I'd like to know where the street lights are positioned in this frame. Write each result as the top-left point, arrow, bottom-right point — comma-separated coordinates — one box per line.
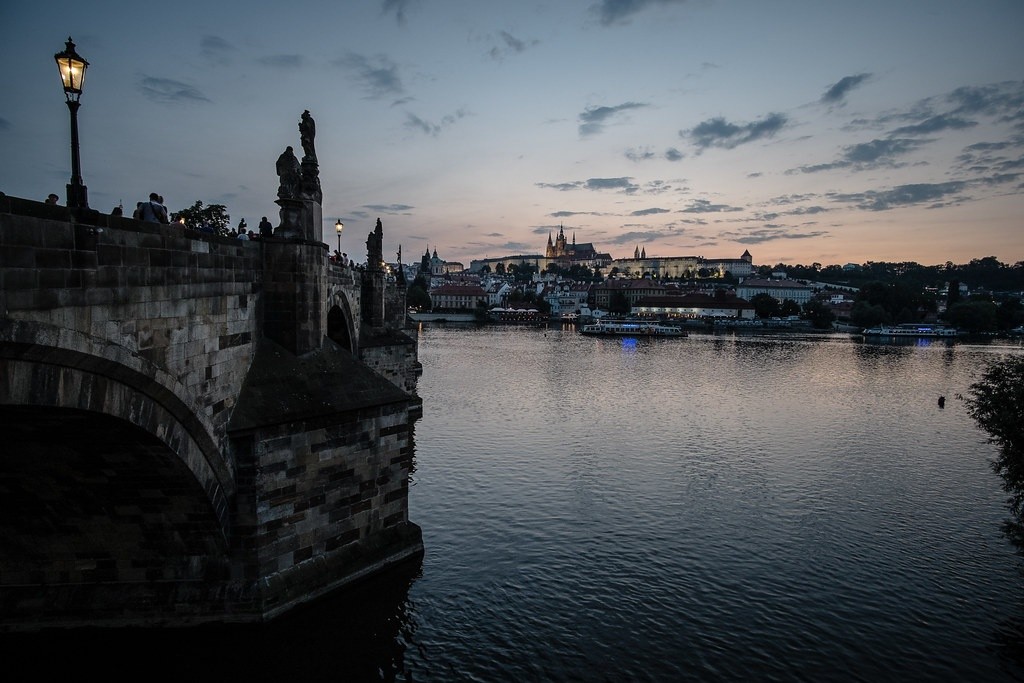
54,35 -> 90,205
334,218 -> 343,256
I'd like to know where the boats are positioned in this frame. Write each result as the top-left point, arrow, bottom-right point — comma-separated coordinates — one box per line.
833,322 -> 858,331
863,323 -> 958,336
583,319 -> 685,336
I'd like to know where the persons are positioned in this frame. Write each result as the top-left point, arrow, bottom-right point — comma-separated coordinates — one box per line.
112,207 -> 122,217
200,222 -> 214,234
229,218 -> 246,237
259,217 -> 272,237
45,194 -> 59,204
333,250 -> 364,270
276,110 -> 319,178
237,229 -> 257,240
374,218 -> 382,233
133,193 -> 182,224
